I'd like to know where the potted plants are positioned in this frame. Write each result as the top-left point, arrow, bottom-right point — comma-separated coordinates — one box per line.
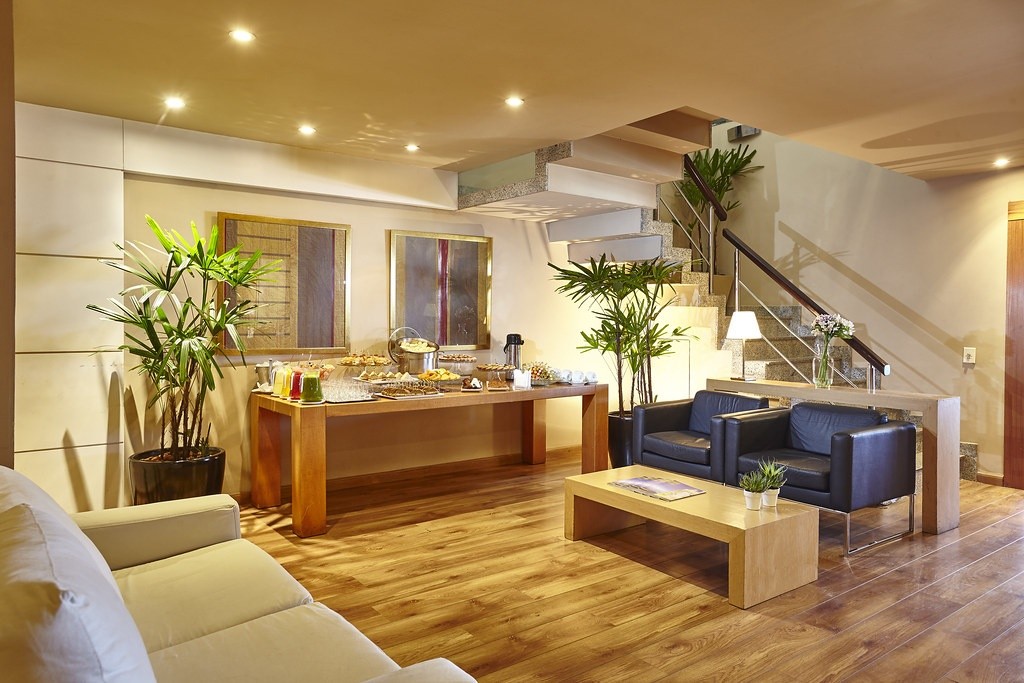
739,470 -> 766,511
757,456 -> 788,507
672,144 -> 765,307
544,255 -> 704,468
86,212 -> 283,504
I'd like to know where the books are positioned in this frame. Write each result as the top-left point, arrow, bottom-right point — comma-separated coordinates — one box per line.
608,476 -> 706,502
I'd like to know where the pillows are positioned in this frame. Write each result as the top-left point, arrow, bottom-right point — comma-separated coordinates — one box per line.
0,465 -> 157,683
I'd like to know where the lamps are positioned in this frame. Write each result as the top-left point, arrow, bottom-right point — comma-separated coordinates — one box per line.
725,310 -> 762,381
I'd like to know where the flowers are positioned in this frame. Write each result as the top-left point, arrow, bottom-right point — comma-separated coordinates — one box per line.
810,311 -> 856,349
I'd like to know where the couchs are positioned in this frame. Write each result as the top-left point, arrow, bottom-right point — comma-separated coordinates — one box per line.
725,401 -> 917,557
633,389 -> 770,482
67,494 -> 478,683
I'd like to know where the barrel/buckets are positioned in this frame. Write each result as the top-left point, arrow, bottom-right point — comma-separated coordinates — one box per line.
254,365 -> 270,387
395,338 -> 440,377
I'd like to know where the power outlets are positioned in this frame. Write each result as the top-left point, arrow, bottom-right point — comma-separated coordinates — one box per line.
963,347 -> 976,363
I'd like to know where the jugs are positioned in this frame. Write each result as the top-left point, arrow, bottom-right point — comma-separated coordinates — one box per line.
270,367 -> 325,404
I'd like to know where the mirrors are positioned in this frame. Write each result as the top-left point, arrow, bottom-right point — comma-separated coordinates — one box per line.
390,229 -> 493,353
215,211 -> 350,356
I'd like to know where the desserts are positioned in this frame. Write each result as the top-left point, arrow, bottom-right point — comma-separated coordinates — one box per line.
341,342 -> 516,396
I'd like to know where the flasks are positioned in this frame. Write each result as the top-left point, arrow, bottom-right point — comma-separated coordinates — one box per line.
503,334 -> 524,381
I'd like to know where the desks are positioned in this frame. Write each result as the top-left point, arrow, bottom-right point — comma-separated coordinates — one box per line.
705,378 -> 961,535
250,381 -> 609,539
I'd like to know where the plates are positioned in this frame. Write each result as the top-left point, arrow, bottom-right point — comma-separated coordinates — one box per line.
461,381 -> 483,391
485,381 -> 509,390
374,392 -> 445,400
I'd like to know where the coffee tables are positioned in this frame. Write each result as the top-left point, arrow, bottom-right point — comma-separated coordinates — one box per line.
565,465 -> 820,610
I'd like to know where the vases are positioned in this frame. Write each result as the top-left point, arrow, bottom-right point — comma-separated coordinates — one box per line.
812,341 -> 835,390
